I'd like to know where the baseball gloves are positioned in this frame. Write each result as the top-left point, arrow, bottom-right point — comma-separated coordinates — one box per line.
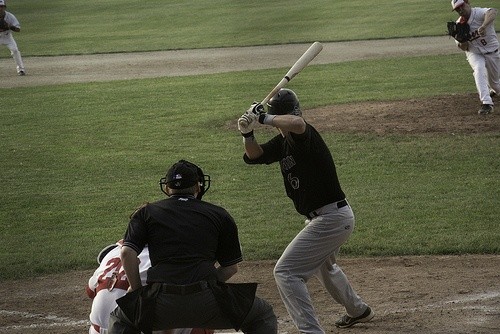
446,21 -> 471,43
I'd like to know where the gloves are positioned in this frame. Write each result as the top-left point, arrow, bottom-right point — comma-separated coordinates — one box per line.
248,100 -> 276,127
238,110 -> 258,138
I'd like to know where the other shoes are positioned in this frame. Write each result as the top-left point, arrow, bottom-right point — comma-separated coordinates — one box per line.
18,71 -> 25,76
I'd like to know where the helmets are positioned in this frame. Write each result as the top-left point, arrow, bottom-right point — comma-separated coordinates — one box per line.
266,88 -> 300,117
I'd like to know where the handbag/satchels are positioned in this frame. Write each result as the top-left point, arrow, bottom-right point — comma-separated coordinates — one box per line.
210,276 -> 258,332
116,282 -> 178,334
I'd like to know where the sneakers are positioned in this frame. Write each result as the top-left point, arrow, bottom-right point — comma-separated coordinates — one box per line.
489,90 -> 500,102
478,105 -> 494,116
334,306 -> 375,328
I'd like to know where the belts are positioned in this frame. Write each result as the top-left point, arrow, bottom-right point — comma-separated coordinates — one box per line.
486,49 -> 498,55
162,280 -> 212,295
94,279 -> 129,294
305,200 -> 348,220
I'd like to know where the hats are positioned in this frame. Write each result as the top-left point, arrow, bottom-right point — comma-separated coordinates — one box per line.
165,162 -> 199,190
0,0 -> 6,6
451,0 -> 466,12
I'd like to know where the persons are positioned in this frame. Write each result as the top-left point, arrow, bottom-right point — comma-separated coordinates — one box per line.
237,87 -> 376,334
87,201 -> 153,334
0,0 -> 25,75
108,160 -> 278,334
450,0 -> 500,115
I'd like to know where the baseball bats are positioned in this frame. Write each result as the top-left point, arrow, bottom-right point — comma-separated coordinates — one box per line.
238,41 -> 324,128
